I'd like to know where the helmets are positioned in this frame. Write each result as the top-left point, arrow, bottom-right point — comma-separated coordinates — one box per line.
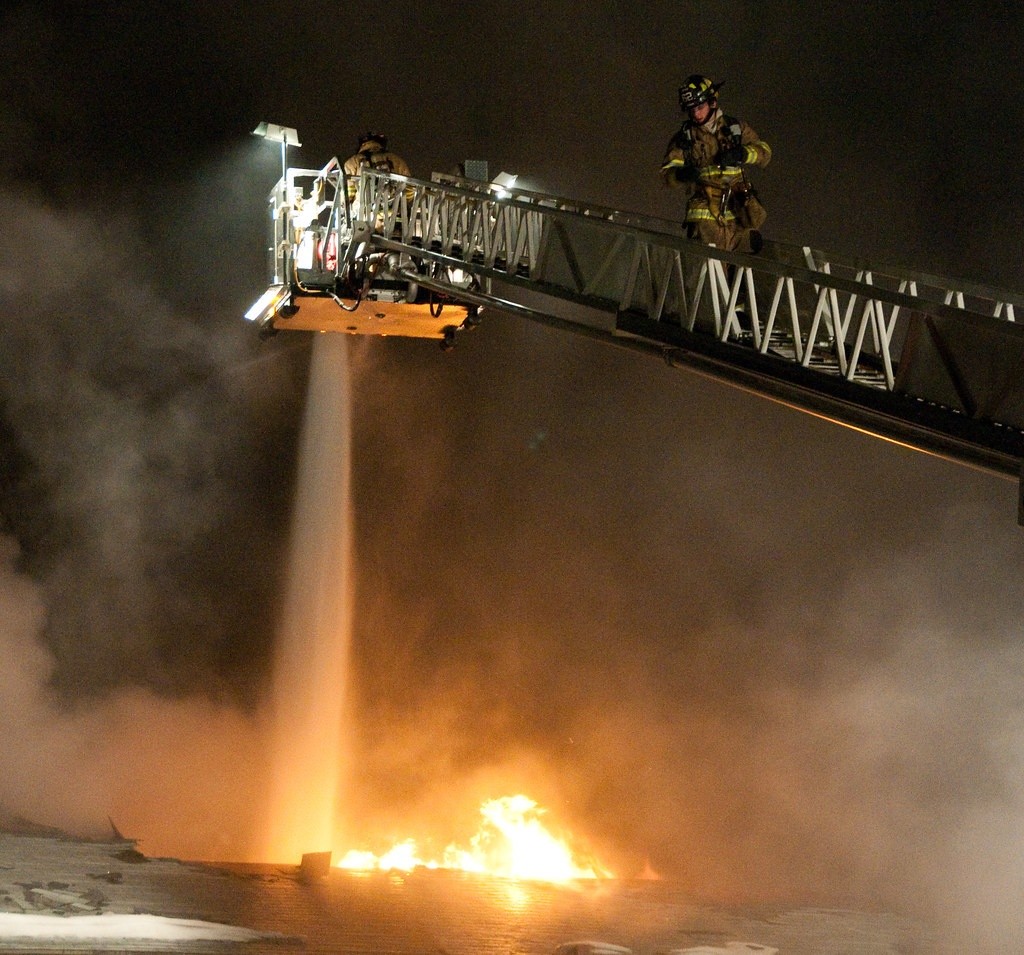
356,128 -> 388,144
678,74 -> 719,112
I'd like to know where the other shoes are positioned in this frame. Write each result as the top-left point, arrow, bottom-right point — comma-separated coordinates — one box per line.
736,311 -> 752,330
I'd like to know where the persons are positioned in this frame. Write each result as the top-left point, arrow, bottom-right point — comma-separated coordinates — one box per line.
659,73 -> 773,340
340,131 -> 418,280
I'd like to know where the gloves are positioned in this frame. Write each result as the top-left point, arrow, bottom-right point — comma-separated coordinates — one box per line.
713,145 -> 748,171
673,166 -> 690,185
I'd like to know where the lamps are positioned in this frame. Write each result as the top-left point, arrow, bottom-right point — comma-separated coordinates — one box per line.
254,121 -> 301,285
491,171 -> 518,188
